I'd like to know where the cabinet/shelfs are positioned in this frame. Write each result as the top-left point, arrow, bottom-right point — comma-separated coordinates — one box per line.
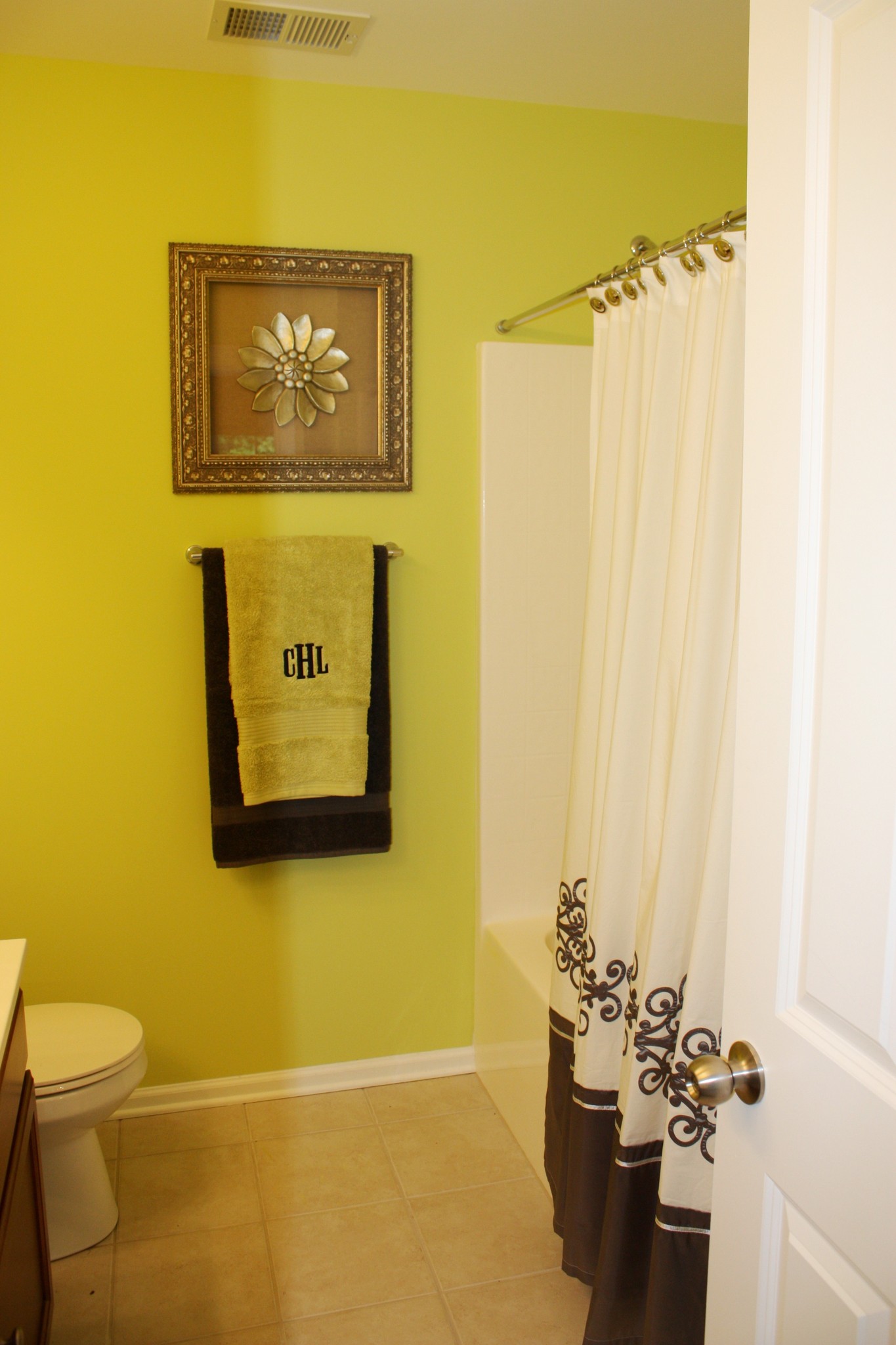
0,937 -> 55,1345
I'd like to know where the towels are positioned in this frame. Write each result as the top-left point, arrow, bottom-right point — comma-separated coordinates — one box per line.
200,535 -> 394,869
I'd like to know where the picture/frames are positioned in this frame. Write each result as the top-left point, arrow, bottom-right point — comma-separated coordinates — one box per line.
168,241 -> 415,494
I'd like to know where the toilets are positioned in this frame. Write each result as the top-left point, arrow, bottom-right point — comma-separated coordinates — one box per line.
22,1003 -> 148,1260
